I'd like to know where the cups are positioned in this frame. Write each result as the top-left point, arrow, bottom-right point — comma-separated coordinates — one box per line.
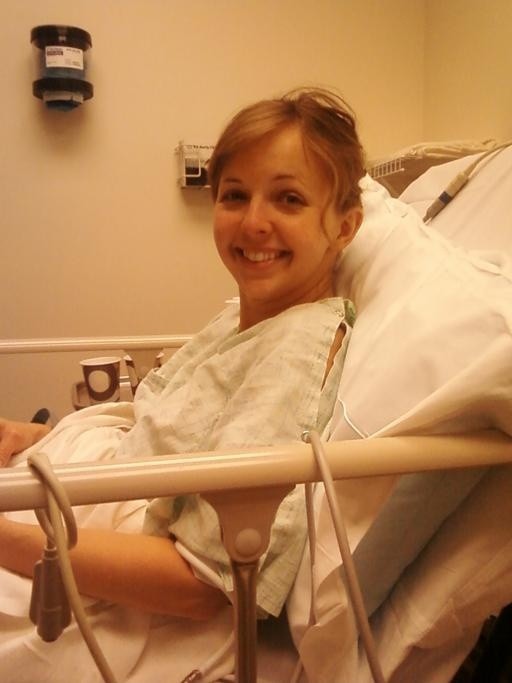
79,352 -> 122,405
124,351 -> 164,403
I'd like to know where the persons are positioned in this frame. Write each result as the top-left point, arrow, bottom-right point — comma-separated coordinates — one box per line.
0,86 -> 368,683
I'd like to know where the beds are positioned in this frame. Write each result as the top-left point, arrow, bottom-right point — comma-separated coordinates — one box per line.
2,142 -> 512,680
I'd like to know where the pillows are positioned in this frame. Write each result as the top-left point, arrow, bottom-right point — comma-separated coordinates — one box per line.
280,167 -> 512,681
